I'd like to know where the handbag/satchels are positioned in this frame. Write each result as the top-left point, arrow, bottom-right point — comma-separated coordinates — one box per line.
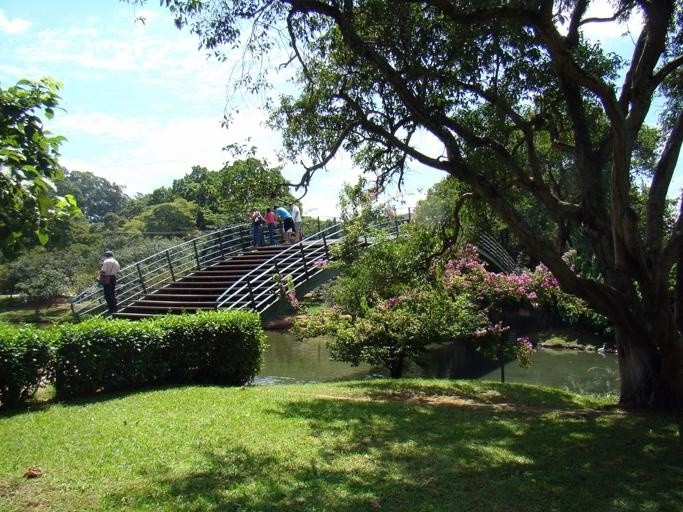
253,216 -> 261,223
96,274 -> 110,285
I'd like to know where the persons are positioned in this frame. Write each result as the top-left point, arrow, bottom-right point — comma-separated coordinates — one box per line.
99,250 -> 121,314
250,202 -> 302,247
387,206 -> 395,222
